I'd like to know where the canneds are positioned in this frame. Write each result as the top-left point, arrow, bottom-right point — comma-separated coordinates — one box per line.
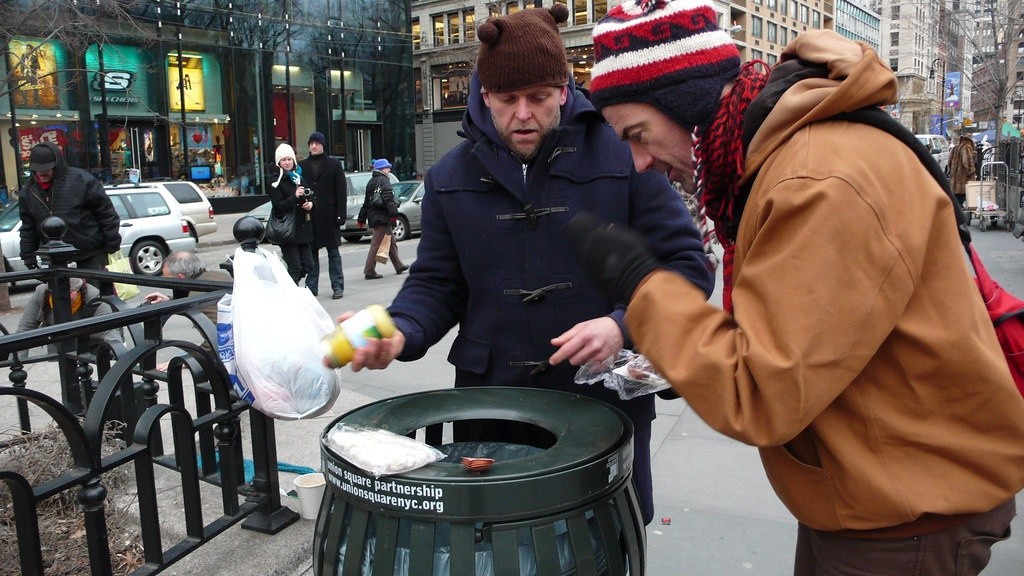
319,304 -> 394,370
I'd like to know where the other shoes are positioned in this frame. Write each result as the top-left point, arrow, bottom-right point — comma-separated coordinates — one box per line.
397,264 -> 409,275
365,272 -> 383,279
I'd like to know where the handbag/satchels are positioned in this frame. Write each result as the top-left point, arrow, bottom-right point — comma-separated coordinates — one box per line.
970,242 -> 1023,396
371,177 -> 400,208
264,203 -> 298,244
106,251 -> 141,301
215,244 -> 339,420
375,223 -> 391,264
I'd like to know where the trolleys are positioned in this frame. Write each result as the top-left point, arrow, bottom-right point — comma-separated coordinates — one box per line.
962,162 -> 1015,234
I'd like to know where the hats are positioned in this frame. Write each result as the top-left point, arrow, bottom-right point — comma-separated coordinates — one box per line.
308,133 -> 325,146
29,149 -> 55,172
271,144 -> 301,188
66,263 -> 83,292
374,159 -> 392,172
589,1 -> 742,271
478,4 -> 570,94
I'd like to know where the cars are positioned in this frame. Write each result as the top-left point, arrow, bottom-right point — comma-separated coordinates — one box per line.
339,179 -> 424,243
100,180 -> 216,242
244,171 -> 400,237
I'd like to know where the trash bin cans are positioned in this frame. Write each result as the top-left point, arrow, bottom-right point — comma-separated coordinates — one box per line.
311,386 -> 647,576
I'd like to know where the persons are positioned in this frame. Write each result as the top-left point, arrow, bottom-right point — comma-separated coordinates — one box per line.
143,251 -> 233,372
570,0 -> 1024,576
13,264 -> 126,382
946,134 -> 975,206
356,159 -> 411,279
19,142 -> 127,352
267,142 -> 315,288
300,133 -> 348,298
322,4 -> 717,524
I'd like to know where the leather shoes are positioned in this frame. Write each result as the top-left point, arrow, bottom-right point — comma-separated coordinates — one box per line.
333,287 -> 343,299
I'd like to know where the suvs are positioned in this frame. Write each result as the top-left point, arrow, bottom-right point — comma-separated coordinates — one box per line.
0,180 -> 197,277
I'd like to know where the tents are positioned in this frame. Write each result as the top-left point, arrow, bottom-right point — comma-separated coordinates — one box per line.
971,121 -> 1020,143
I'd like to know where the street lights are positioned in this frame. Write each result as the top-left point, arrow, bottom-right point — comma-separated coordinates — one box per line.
930,58 -> 945,133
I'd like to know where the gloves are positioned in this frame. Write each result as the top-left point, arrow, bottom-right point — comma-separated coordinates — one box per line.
26,263 -> 46,283
357,221 -> 363,228
560,207 -> 659,309
337,217 -> 345,226
390,217 -> 397,228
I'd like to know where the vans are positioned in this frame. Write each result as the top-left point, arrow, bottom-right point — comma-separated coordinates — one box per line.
914,133 -> 951,176
